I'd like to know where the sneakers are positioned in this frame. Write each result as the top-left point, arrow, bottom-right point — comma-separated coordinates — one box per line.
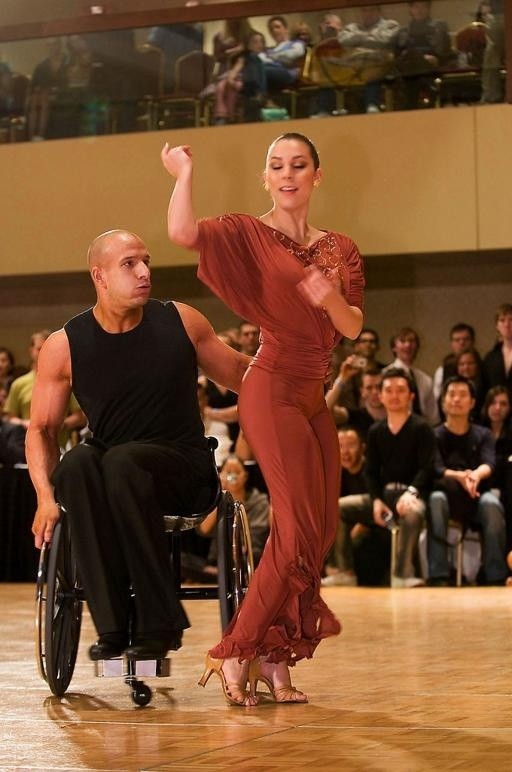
322,575 -> 358,585
392,576 -> 425,589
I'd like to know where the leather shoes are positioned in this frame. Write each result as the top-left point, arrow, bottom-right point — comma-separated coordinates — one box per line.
428,576 -> 453,586
125,628 -> 184,659
89,627 -> 132,659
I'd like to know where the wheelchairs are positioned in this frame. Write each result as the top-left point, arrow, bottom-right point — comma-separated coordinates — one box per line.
25,428 -> 265,711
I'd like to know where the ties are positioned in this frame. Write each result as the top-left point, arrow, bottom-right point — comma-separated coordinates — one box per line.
408,367 -> 423,416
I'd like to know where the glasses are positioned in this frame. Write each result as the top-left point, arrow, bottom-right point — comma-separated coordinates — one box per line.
357,339 -> 377,345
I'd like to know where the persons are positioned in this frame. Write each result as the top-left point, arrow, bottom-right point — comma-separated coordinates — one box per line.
0,4 -> 512,142
2,304 -> 511,593
159,132 -> 368,708
23,227 -> 336,662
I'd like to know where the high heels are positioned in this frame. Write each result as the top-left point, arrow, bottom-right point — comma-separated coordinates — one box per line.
248,656 -> 309,704
198,653 -> 262,708
